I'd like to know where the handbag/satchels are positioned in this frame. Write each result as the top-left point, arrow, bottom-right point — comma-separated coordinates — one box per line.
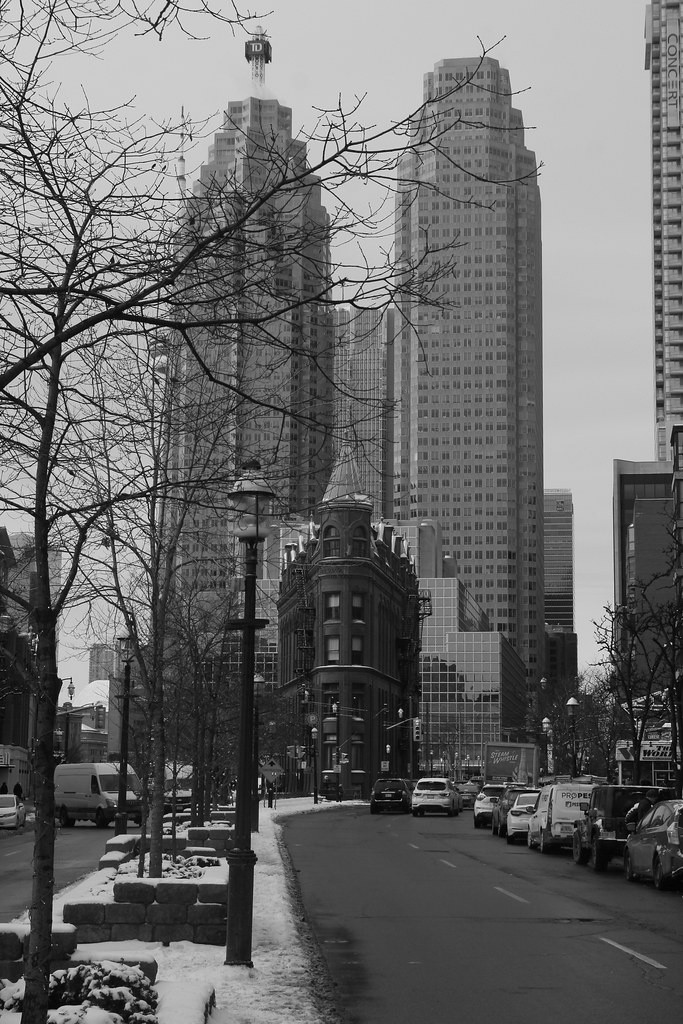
339,792 -> 343,795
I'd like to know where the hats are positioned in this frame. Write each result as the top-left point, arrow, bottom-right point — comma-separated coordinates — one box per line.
339,784 -> 342,786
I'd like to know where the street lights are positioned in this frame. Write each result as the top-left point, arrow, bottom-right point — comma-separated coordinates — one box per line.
250,668 -> 267,831
226,456 -> 280,967
113,629 -> 134,837
442,750 -> 447,778
312,726 -> 319,805
430,749 -> 434,778
466,754 -> 471,779
477,755 -> 481,776
565,697 -> 580,780
454,751 -> 459,781
542,717 -> 551,775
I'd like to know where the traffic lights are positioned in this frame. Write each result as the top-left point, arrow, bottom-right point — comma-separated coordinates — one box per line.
412,718 -> 422,742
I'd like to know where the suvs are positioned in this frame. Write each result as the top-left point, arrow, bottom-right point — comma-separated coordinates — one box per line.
573,783 -> 680,873
526,774 -> 612,856
369,777 -> 416,814
474,784 -> 519,828
411,778 -> 463,817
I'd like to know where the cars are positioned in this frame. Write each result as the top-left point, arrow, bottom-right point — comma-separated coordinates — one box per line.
447,776 -> 529,793
504,792 -> 543,843
489,785 -> 541,838
458,784 -> 481,808
0,794 -> 26,831
623,799 -> 683,891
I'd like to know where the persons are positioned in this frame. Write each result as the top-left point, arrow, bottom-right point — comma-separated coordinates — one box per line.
0,782 -> 8,794
13,782 -> 22,799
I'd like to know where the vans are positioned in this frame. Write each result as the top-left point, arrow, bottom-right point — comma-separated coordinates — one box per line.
55,762 -> 151,829
162,762 -> 194,817
